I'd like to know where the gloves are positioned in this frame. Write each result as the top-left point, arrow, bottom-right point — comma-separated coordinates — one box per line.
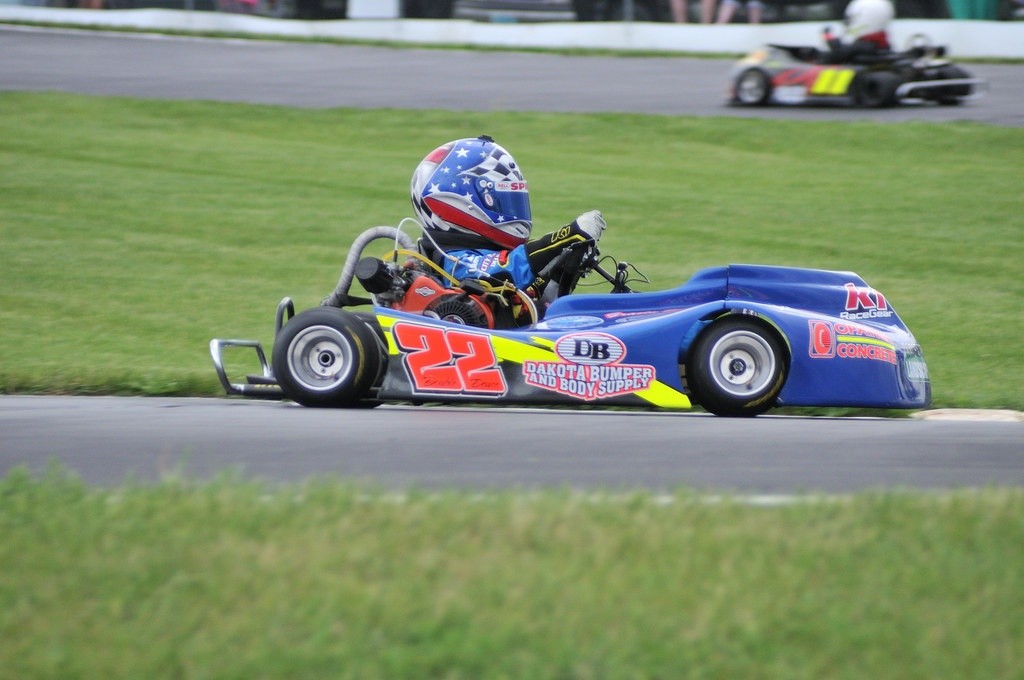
526,210 -> 606,291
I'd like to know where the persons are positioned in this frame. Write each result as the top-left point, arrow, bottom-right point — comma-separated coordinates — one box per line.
825,0 -> 894,61
671,0 -> 766,24
410,135 -> 605,326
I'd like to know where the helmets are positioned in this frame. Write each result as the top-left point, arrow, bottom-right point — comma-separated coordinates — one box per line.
410,135 -> 533,250
845,0 -> 894,39
